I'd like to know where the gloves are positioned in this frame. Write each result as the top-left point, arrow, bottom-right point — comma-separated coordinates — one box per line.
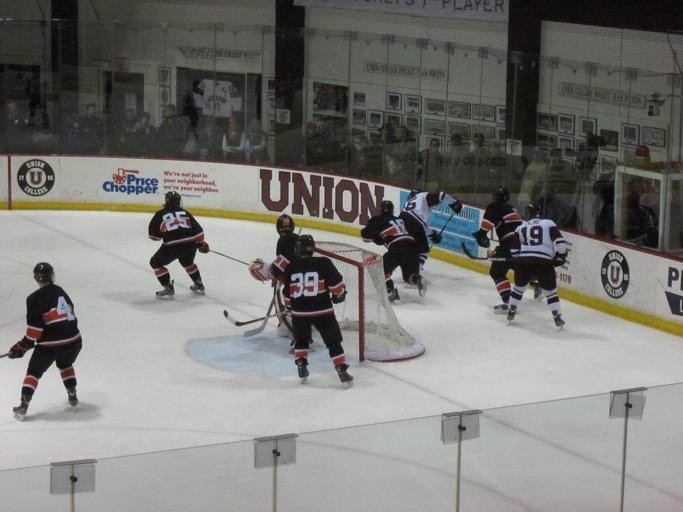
331,291 -> 347,303
199,241 -> 208,253
249,258 -> 270,282
477,236 -> 490,247
428,230 -> 441,244
7,341 -> 27,359
448,196 -> 462,214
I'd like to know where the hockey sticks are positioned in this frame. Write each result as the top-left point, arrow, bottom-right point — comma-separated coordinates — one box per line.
460,242 -> 488,262
244,226 -> 303,337
224,291 -> 349,327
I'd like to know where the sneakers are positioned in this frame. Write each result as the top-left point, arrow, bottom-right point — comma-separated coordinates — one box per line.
388,289 -> 399,302
494,304 -> 508,311
507,309 -> 516,319
155,279 -> 175,296
295,358 -> 308,377
554,316 -> 565,327
13,403 -> 28,415
190,279 -> 204,290
335,363 -> 353,382
417,277 -> 427,297
67,391 -> 79,406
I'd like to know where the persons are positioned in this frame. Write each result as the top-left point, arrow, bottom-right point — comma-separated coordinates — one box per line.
471,186 -> 543,315
248,214 -> 315,356
1,100 -> 682,252
507,203 -> 570,330
283,234 -> 353,389
147,191 -> 209,299
7,261 -> 82,420
360,200 -> 428,301
399,186 -> 462,273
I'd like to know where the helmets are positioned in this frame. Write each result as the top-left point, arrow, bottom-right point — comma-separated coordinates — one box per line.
380,201 -> 394,215
276,214 -> 294,232
525,203 -> 543,218
165,192 -> 181,203
296,235 -> 315,252
493,187 -> 510,201
410,188 -> 421,197
33,263 -> 52,282
